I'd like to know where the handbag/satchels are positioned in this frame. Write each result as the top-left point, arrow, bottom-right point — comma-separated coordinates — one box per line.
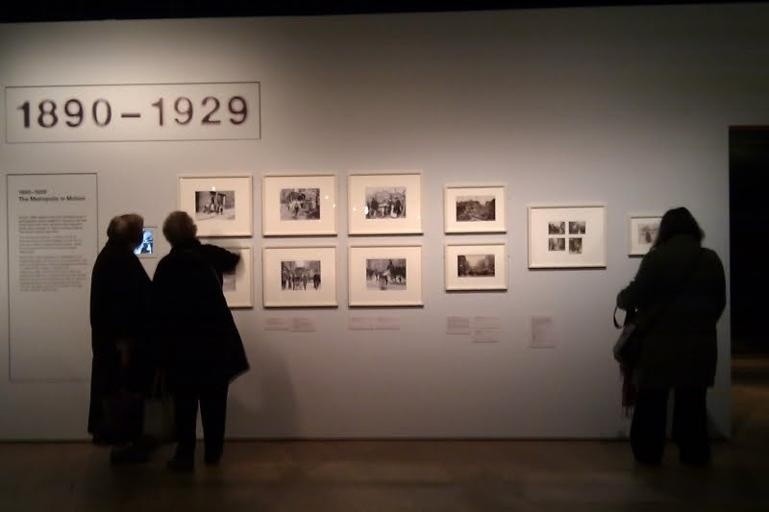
613,316 -> 647,362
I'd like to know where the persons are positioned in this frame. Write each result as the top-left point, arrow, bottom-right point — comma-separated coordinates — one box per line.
152,211 -> 250,473
87,214 -> 157,469
282,266 -> 322,291
366,260 -> 407,288
365,195 -> 408,219
615,207 -> 726,471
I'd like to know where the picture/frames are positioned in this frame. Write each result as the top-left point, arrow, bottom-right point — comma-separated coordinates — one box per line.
629,214 -> 660,256
263,245 -> 339,308
177,176 -> 253,237
347,171 -> 426,236
524,203 -> 608,269
444,185 -> 507,234
347,242 -> 425,307
442,242 -> 508,291
220,246 -> 256,310
259,173 -> 340,236
